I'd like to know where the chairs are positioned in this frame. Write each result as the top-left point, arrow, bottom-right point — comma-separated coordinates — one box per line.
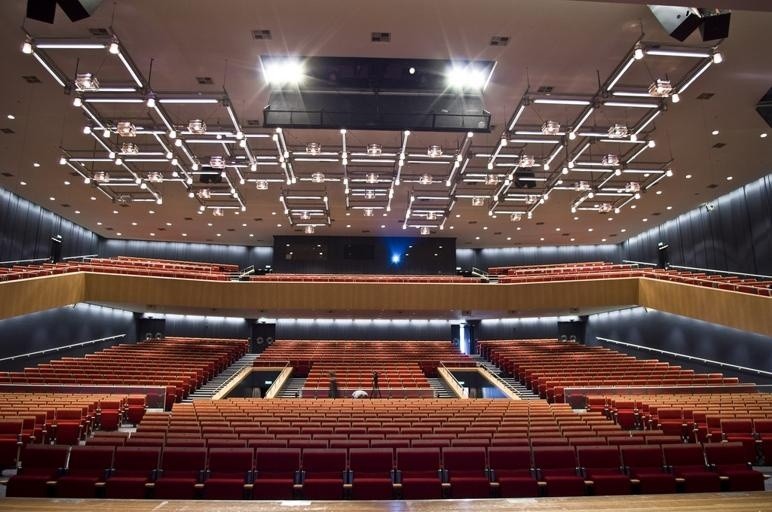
639,264 -> 772,338
0,333 -> 771,500
0,253 -> 642,316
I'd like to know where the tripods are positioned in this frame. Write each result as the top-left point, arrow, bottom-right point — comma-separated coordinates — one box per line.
370,380 -> 382,399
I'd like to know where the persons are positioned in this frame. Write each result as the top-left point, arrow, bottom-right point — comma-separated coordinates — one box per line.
330,370 -> 338,398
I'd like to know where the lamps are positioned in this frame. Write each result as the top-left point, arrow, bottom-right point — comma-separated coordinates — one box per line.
19,39 -> 722,235
